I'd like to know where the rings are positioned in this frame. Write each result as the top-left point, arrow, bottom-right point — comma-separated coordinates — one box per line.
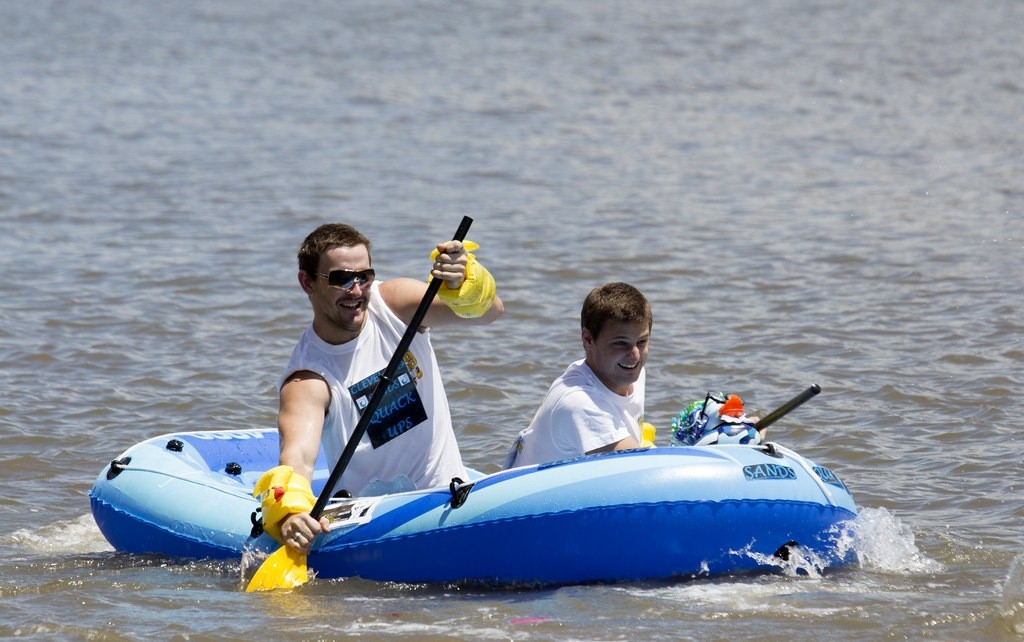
294,532 -> 301,541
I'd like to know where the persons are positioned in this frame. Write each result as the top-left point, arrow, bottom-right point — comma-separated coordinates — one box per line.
506,281 -> 654,468
273,222 -> 508,556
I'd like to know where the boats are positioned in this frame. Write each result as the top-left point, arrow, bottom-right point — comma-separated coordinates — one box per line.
86,427 -> 861,594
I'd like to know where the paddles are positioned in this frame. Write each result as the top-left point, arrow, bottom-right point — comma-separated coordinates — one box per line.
243,213 -> 476,595
752,382 -> 822,432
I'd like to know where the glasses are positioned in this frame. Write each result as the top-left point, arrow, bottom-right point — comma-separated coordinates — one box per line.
313,267 -> 375,291
